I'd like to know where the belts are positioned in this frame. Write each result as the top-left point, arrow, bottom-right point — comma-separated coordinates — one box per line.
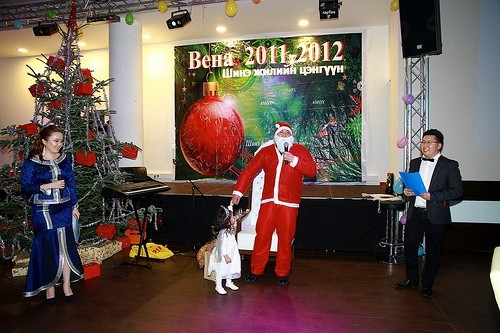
413,207 -> 427,213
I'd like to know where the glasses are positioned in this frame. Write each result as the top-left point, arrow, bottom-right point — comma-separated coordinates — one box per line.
420,139 -> 440,147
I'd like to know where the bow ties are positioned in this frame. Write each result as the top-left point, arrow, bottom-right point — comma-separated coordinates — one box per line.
422,157 -> 434,162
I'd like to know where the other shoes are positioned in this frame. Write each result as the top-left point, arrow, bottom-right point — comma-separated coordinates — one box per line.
245,273 -> 264,283
214,287 -> 227,295
225,282 -> 239,290
277,277 -> 289,285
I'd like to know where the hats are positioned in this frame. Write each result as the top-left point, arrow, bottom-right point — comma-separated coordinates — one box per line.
274,122 -> 293,136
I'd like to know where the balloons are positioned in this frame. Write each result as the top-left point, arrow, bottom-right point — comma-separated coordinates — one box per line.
418,247 -> 424,257
157,0 -> 168,14
393,178 -> 404,194
13,20 -> 23,30
397,137 -> 408,149
405,94 -> 414,104
125,11 -> 135,25
225,0 -> 237,17
390,0 -> 399,10
252,0 -> 261,4
400,214 -> 407,224
45,8 -> 57,19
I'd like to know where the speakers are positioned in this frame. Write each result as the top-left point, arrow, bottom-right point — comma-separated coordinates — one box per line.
398,0 -> 442,58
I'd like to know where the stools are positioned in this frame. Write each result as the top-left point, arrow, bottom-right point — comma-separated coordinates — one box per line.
379,201 -> 406,265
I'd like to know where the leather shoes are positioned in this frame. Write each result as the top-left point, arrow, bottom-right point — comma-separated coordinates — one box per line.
395,279 -> 419,289
422,287 -> 433,299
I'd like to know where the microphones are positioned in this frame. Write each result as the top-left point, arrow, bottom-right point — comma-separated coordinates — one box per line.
284,142 -> 288,164
58,175 -> 63,197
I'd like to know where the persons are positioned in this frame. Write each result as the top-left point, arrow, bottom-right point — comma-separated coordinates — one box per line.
207,200 -> 241,294
400,128 -> 465,298
231,121 -> 316,285
22,124 -> 86,305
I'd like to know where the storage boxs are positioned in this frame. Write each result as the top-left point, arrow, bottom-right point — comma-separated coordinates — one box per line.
122,145 -> 138,159
28,83 -> 50,97
118,236 -> 130,248
76,151 -> 96,166
81,70 -> 93,83
47,56 -> 65,70
47,100 -> 66,112
82,262 -> 101,280
96,224 -> 117,239
73,83 -> 92,96
17,123 -> 38,137
130,219 -> 147,230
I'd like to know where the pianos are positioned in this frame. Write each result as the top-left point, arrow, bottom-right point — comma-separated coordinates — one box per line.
101,180 -> 171,270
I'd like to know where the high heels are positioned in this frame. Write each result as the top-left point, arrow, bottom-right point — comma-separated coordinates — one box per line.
64,294 -> 75,302
46,297 -> 56,306
